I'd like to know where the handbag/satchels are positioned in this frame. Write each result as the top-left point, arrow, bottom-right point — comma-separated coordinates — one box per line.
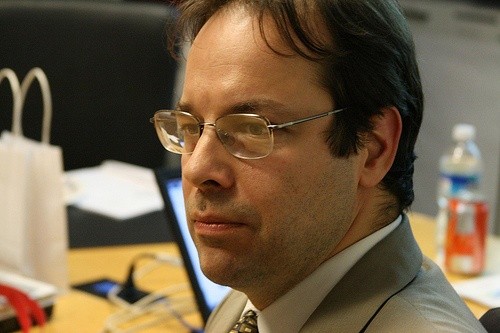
0,68 -> 68,321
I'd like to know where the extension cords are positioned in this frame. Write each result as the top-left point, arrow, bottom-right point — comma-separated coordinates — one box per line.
106,283 -> 169,315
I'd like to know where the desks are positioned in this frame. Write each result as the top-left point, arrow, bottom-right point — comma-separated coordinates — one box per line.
12,209 -> 500,333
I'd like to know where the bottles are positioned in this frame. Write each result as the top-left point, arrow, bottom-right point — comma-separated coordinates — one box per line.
436,124 -> 480,270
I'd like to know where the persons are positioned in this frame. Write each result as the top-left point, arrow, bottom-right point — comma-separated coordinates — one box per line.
166,0 -> 487,333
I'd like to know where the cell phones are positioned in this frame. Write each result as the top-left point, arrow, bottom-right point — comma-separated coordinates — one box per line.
72,277 -> 120,301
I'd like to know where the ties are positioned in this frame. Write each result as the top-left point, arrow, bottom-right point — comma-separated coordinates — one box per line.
227,311 -> 259,333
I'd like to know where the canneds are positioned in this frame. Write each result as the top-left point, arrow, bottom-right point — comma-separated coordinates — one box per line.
443,191 -> 489,276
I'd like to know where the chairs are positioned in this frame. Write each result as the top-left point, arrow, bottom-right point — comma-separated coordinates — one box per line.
0,1 -> 192,179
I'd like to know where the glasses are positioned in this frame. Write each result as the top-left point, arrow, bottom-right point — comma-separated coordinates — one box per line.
149,105 -> 348,160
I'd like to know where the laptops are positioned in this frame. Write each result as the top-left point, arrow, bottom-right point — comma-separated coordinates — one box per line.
154,163 -> 233,324
